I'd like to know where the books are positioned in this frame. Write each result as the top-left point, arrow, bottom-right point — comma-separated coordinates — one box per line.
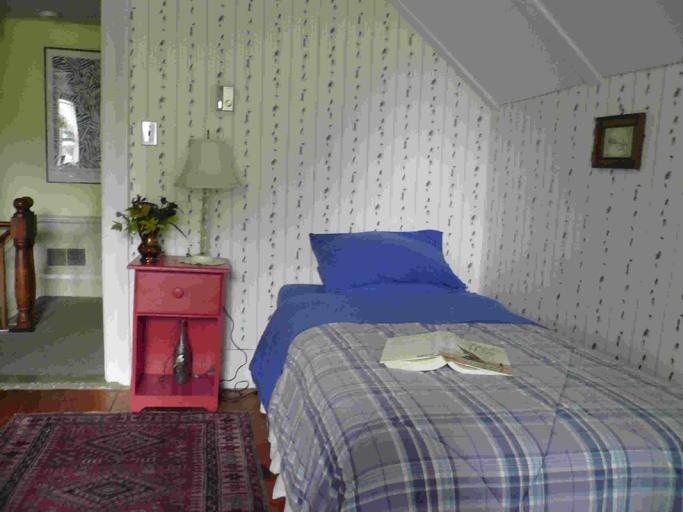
380,330 -> 514,379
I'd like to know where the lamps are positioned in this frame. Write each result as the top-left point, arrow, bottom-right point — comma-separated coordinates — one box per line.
173,140 -> 242,267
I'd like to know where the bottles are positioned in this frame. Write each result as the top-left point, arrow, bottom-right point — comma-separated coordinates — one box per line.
172,319 -> 191,383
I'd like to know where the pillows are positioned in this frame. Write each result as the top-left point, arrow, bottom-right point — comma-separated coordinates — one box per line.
309,229 -> 466,289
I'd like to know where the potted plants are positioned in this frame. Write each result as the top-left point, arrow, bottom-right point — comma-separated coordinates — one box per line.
109,195 -> 179,264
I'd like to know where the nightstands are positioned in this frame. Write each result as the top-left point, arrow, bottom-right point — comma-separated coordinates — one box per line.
125,252 -> 232,413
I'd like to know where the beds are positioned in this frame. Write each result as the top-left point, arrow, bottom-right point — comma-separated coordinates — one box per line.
276,284 -> 683,510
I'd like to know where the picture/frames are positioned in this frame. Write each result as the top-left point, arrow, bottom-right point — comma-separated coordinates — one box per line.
43,45 -> 101,185
591,111 -> 645,169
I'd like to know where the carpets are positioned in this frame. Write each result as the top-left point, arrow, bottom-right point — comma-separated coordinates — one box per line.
0,412 -> 265,512
